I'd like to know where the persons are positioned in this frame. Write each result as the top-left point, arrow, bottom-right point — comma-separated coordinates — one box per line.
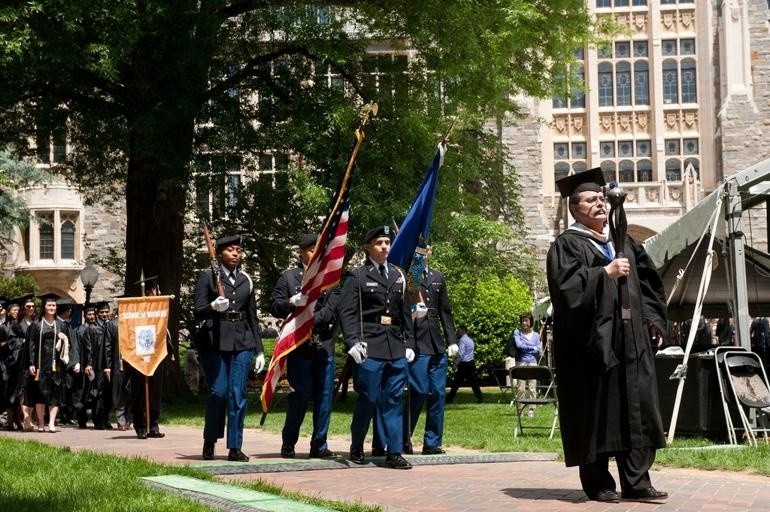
270,235 -> 341,458
403,238 -> 459,454
339,226 -> 416,469
673,314 -> 770,360
193,236 -> 265,462
0,276 -> 175,439
513,313 -> 542,416
371,384 -> 413,458
546,165 -> 668,503
446,325 -> 484,403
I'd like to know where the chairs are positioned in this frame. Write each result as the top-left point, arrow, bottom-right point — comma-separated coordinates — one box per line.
508,364 -> 563,440
712,341 -> 769,448
722,348 -> 769,450
492,368 -> 520,404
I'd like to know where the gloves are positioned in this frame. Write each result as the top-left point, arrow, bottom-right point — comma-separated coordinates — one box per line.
415,301 -> 428,319
405,346 -> 416,363
347,341 -> 369,366
253,350 -> 266,375
447,343 -> 459,357
210,294 -> 230,313
288,291 -> 309,307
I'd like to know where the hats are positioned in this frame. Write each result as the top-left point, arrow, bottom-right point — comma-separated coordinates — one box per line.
215,234 -> 242,252
555,166 -> 607,199
0,274 -> 161,311
363,226 -> 395,245
300,234 -> 318,250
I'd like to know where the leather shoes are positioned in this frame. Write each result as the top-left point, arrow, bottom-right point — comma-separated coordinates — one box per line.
349,445 -> 366,465
227,448 -> 250,462
620,486 -> 670,504
308,448 -> 338,458
385,453 -> 414,470
372,440 -> 448,456
280,443 -> 295,457
203,440 -> 214,459
6,418 -> 166,439
581,484 -> 622,505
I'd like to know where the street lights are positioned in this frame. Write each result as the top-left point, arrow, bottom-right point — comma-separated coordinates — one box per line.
80,259 -> 99,323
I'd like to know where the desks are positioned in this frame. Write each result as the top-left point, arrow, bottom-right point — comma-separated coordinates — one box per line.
654,353 -> 721,437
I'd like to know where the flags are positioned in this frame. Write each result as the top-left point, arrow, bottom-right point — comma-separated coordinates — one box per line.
386,142 -> 449,269
259,129 -> 366,417
